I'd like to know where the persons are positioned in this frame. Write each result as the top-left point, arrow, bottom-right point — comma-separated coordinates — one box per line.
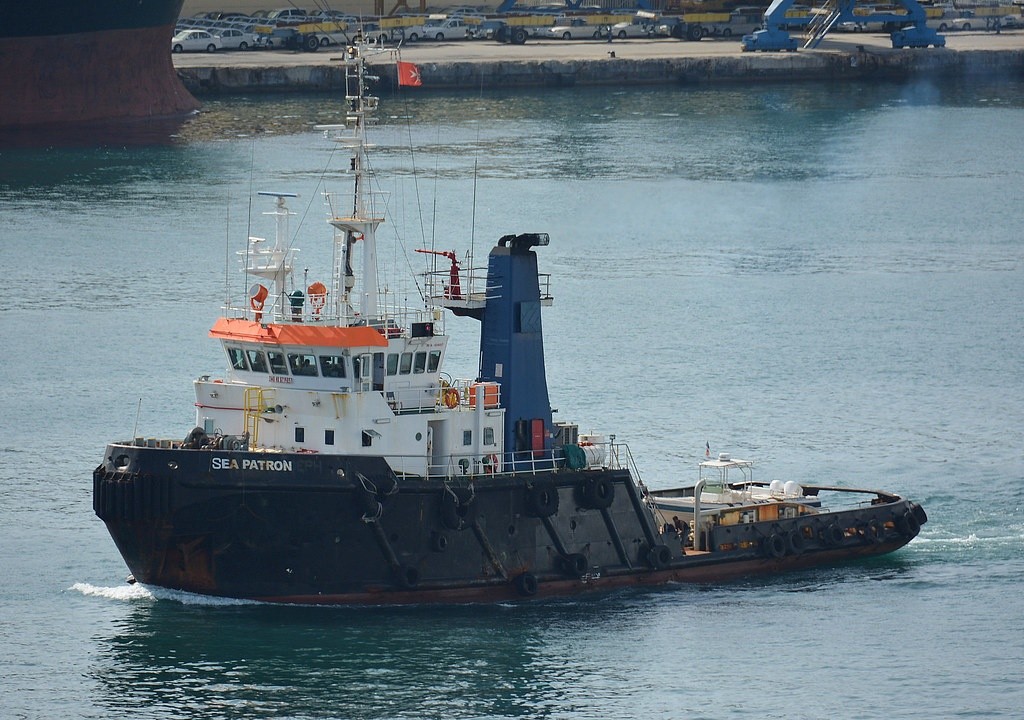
672,516 -> 690,546
229,348 -> 344,377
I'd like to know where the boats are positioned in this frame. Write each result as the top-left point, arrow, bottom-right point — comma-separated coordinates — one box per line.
90,27 -> 929,612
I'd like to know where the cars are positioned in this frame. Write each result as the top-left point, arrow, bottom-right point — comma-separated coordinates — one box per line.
170,0 -> 1024,60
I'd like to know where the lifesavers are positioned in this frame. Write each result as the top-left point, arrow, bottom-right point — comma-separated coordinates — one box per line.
783,528 -> 806,554
650,545 -> 671,567
446,389 -> 459,409
484,454 -> 498,473
565,554 -> 588,574
589,477 -> 614,508
435,531 -> 449,552
763,533 -> 785,558
531,482 -> 559,517
823,524 -> 845,545
516,572 -> 537,596
910,502 -> 927,525
864,518 -> 887,544
902,511 -> 920,536
397,561 -> 422,589
